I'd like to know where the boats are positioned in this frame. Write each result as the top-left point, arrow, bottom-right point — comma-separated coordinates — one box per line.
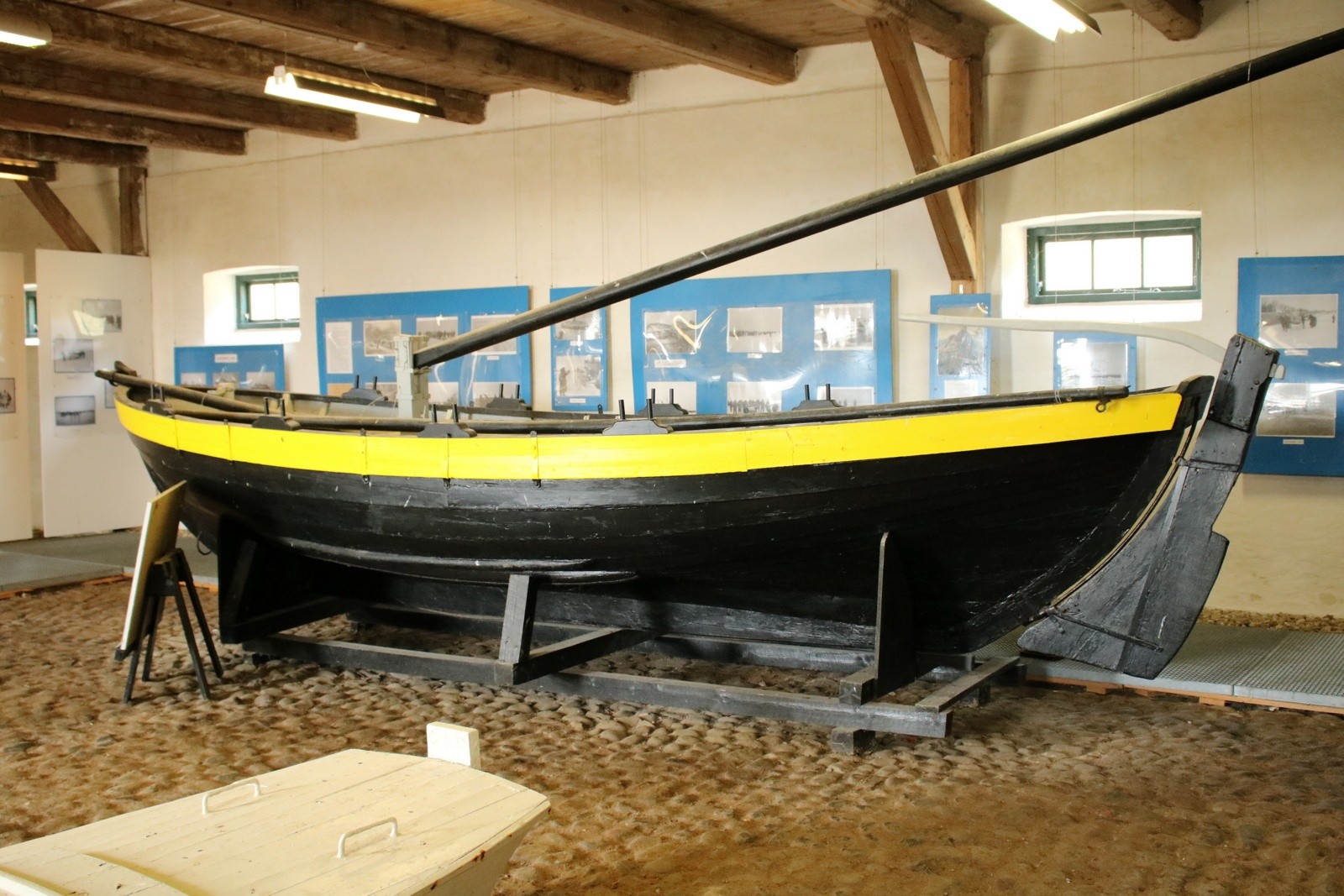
95,333 -> 1288,761
0,722 -> 552,896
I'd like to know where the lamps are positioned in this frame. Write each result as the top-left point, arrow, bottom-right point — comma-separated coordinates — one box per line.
266,33 -> 438,123
0,13 -> 53,47
1,153 -> 59,182
986,0 -> 1104,42
